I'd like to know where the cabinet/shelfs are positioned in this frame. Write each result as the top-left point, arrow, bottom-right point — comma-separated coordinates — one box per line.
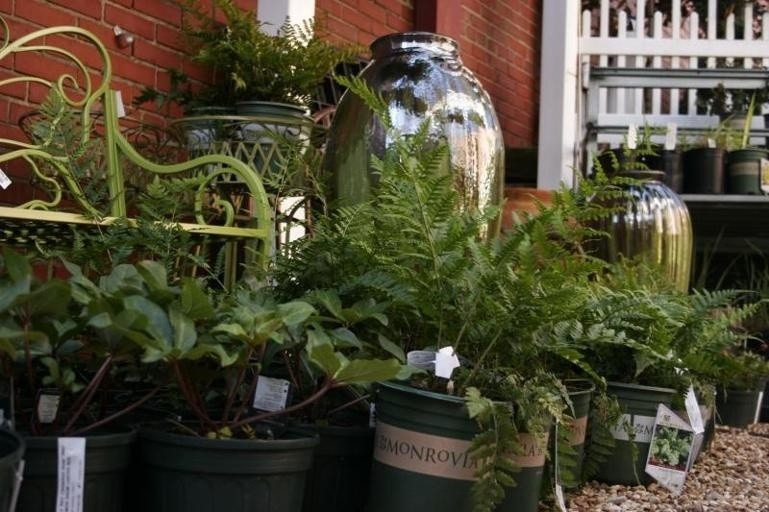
0,14 -> 277,296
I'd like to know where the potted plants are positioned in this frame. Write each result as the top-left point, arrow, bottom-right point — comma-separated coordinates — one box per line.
681,116 -> 727,194
221,5 -> 369,185
143,255 -> 427,511
320,63 -> 660,511
585,289 -> 680,486
721,352 -> 767,426
125,2 -> 235,182
641,129 -> 682,194
729,94 -> 768,195
0,239 -> 220,511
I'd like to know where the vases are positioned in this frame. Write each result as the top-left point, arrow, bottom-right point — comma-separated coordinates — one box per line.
581,170 -> 693,297
505,187 -> 574,263
598,147 -> 637,184
329,32 -> 506,251
